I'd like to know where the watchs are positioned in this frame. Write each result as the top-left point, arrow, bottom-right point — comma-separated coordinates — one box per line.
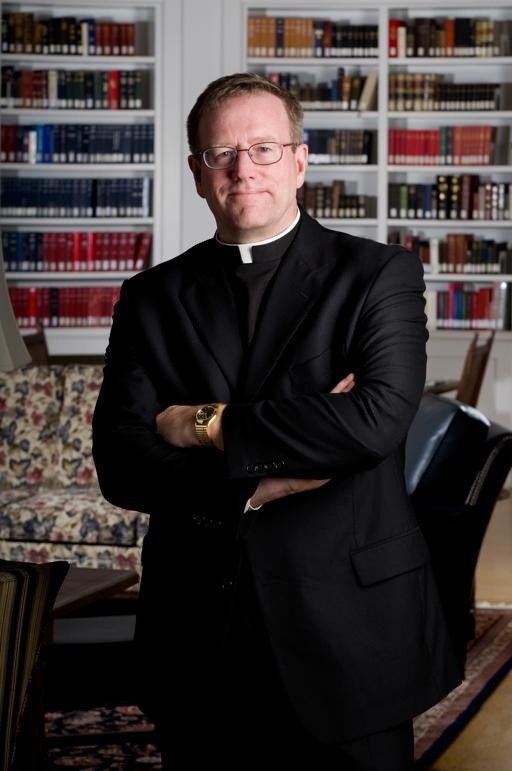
192,401 -> 220,447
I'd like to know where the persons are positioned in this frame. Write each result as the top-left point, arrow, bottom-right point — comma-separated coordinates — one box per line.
91,73 -> 429,771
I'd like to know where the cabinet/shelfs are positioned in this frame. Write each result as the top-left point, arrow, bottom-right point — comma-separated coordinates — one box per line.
219,1 -> 384,249
2,5 -> 168,339
385,4 -> 512,336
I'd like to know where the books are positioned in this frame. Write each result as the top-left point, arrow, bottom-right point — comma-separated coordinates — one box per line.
387,125 -> 511,222
388,228 -> 512,332
246,15 -> 380,112
1,122 -> 155,217
0,10 -> 150,110
388,16 -> 512,109
297,130 -> 378,219
0,230 -> 152,333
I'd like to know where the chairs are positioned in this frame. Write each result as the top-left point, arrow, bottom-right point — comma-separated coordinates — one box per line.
399,370 -> 512,708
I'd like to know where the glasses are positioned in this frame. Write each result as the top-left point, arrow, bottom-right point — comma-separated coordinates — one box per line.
193,142 -> 294,170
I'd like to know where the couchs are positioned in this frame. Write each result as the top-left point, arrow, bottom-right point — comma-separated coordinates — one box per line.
0,350 -> 185,570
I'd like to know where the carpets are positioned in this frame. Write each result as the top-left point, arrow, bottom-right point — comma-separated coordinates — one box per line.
34,580 -> 511,762
